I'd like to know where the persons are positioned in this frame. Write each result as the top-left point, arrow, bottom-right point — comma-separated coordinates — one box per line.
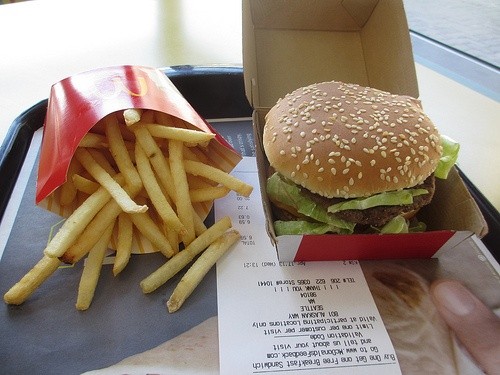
427,272 -> 499,374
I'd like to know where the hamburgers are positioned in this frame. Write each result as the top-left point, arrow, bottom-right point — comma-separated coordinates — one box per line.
262,80 -> 461,236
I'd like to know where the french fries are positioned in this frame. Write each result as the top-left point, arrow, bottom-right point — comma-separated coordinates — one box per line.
5,109 -> 253,313
